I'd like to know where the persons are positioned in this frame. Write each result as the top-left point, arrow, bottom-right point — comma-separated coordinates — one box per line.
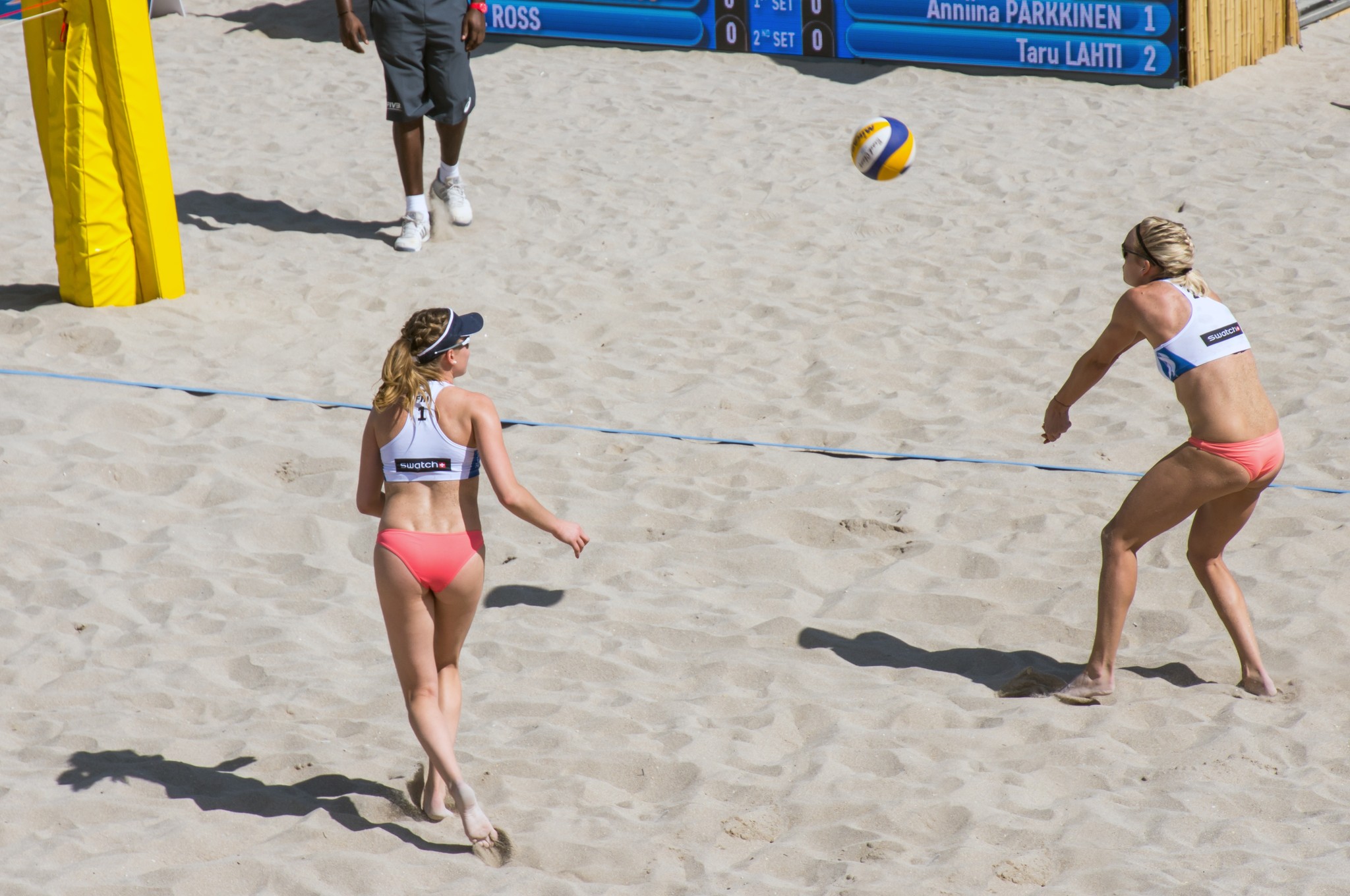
355,308 -> 589,851
335,0 -> 489,253
1042,215 -> 1284,699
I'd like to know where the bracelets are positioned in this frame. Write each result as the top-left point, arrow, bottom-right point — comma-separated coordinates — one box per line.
338,10 -> 354,18
1054,395 -> 1072,407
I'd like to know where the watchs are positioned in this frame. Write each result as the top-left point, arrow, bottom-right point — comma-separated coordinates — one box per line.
468,2 -> 488,14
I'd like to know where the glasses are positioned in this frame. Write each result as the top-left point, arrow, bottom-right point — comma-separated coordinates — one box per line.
435,336 -> 470,354
1122,243 -> 1157,267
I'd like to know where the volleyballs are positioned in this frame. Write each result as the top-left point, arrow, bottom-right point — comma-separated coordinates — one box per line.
850,115 -> 917,182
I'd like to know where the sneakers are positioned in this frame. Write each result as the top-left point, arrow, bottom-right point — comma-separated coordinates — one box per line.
394,210 -> 432,252
432,167 -> 473,227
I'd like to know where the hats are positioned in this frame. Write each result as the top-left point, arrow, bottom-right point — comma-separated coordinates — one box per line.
410,307 -> 483,363
